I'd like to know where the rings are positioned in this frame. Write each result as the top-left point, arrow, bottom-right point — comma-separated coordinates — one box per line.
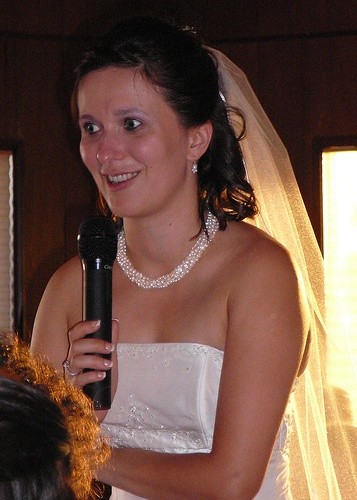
62,359 -> 77,377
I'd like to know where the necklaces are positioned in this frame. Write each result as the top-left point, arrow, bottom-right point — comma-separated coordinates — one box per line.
116,211 -> 221,290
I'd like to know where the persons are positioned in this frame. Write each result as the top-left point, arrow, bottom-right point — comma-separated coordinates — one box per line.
28,19 -> 340,500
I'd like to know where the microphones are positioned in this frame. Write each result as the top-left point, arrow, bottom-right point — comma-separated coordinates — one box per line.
76,216 -> 118,412
